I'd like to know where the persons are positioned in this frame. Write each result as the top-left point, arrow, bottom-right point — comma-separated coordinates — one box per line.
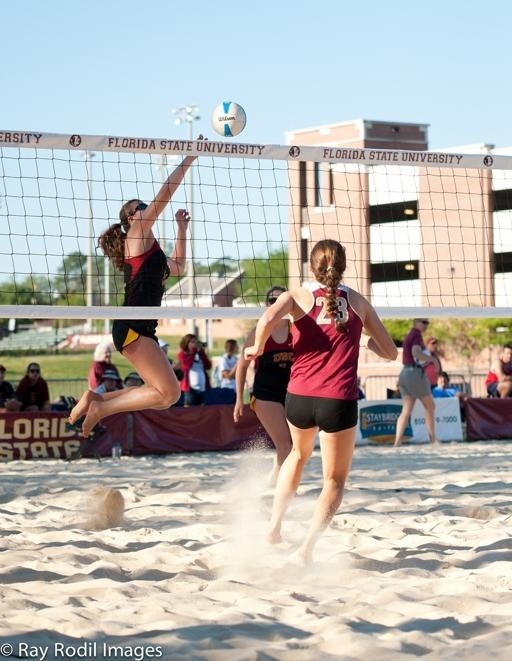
393,318 -> 438,448
68,134 -> 208,439
233,287 -> 294,489
244,239 -> 398,565
0,335 -> 512,412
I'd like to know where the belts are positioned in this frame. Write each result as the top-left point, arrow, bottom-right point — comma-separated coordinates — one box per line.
404,364 -> 421,368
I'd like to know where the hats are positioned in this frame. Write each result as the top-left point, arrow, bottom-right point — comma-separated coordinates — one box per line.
124,372 -> 144,384
101,369 -> 122,383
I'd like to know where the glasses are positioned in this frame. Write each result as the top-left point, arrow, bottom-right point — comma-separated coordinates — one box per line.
268,298 -> 277,304
131,203 -> 148,215
30,369 -> 39,373
422,320 -> 429,324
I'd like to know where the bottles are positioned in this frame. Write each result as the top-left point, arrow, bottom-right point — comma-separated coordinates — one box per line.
112,442 -> 122,467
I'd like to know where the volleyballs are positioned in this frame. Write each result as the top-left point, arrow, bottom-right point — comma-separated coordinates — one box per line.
212,102 -> 245,136
